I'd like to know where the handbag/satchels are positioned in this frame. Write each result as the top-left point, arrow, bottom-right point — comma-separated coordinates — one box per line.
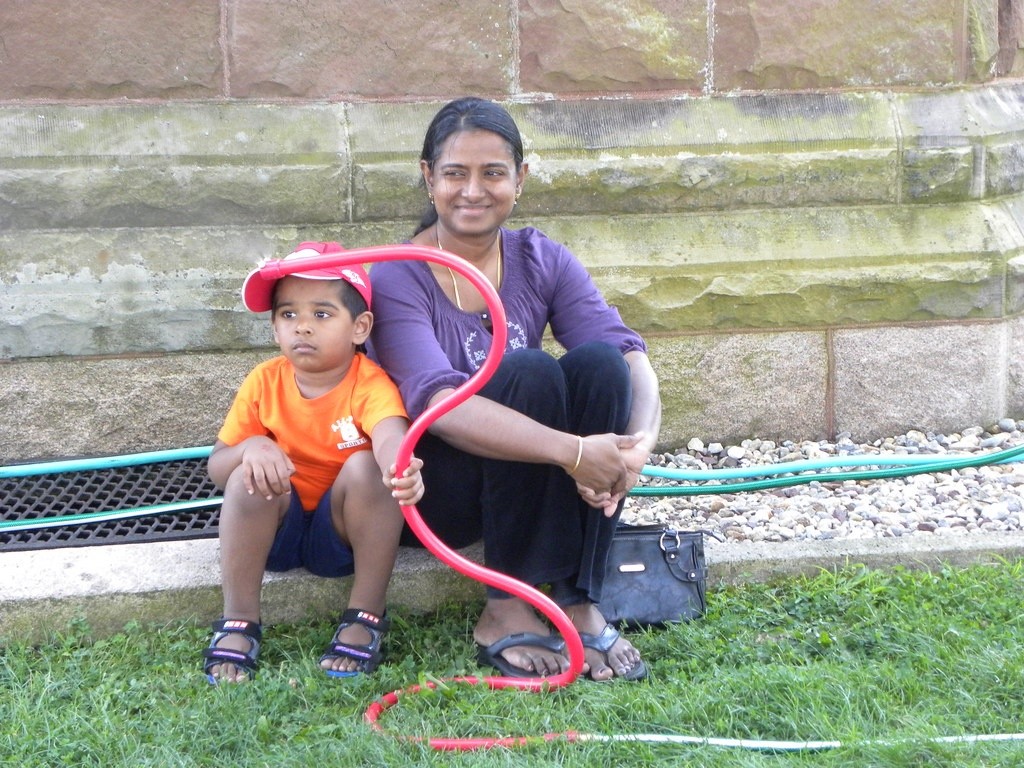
596,523 -> 709,626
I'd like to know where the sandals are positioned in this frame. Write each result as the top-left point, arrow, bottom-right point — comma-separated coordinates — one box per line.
318,606 -> 391,678
202,614 -> 263,685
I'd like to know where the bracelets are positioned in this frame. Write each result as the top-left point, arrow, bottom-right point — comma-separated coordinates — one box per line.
568,434 -> 585,477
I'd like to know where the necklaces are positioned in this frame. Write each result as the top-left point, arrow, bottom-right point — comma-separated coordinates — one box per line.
432,218 -> 501,312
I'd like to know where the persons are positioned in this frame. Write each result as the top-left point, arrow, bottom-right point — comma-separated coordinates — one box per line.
367,98 -> 663,683
199,242 -> 427,684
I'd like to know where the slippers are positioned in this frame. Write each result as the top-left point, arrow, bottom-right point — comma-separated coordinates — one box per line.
576,619 -> 647,683
475,632 -> 572,681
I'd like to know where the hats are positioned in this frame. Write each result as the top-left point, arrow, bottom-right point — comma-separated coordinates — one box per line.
241,241 -> 372,312
